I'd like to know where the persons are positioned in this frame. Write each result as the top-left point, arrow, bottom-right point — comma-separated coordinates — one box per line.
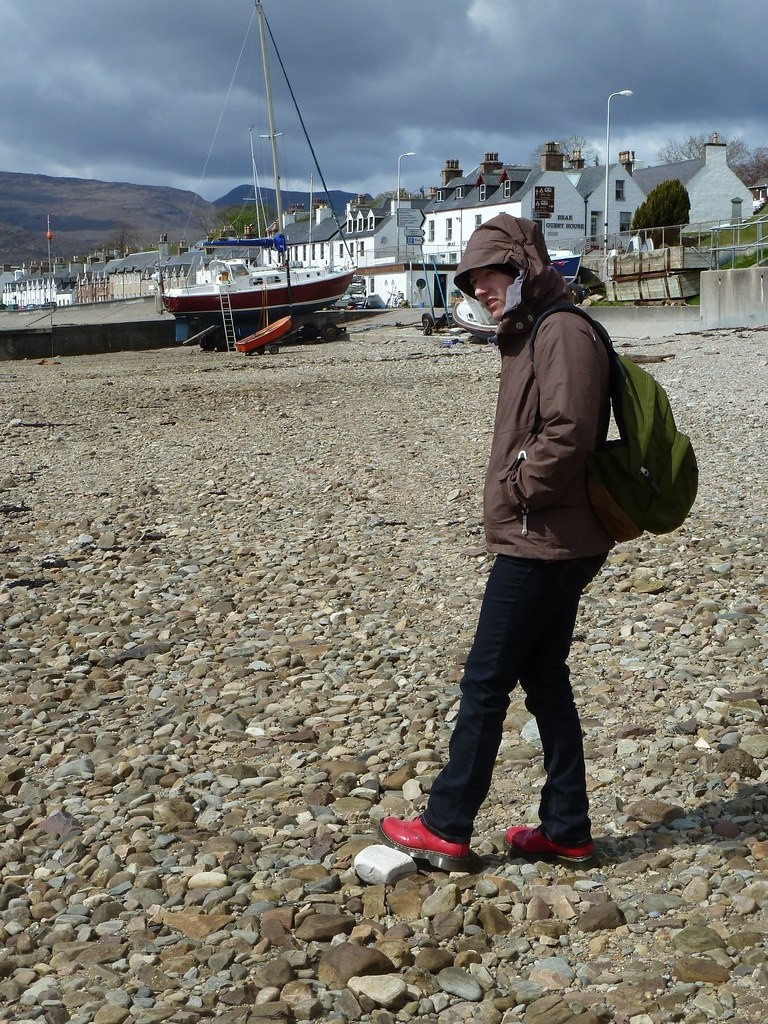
379,214 -> 610,871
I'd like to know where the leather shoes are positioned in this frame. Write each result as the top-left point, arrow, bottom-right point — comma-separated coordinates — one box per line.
377,814 -> 470,872
504,824 -> 597,871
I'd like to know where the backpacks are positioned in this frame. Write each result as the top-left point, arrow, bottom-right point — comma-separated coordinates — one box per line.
528,302 -> 699,544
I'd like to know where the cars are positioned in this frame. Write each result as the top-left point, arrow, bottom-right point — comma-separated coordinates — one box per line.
0,302 -> 57,310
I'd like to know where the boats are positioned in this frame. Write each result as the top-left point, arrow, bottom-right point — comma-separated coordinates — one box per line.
235,315 -> 292,353
603,235 -> 654,282
546,248 -> 582,284
453,290 -> 501,341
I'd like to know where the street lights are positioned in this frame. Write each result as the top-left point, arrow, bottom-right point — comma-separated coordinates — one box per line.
396,152 -> 415,262
604,90 -> 633,256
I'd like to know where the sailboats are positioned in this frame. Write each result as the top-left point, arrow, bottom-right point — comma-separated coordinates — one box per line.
157,0 -> 360,338
